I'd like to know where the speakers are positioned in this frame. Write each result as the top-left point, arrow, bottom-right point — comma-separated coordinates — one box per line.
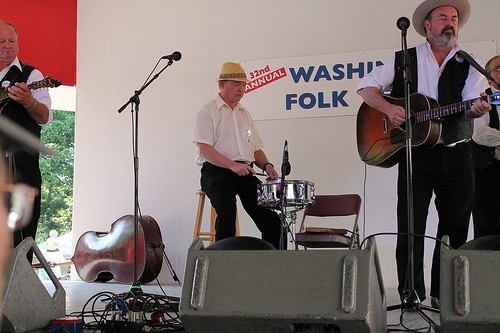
2,236 -> 66,333
180,236 -> 388,333
438,235 -> 500,333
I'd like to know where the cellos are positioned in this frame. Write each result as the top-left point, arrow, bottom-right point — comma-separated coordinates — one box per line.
31,214 -> 182,285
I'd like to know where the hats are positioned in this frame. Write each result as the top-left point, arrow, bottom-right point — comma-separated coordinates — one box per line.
412,0 -> 471,38
219,62 -> 250,82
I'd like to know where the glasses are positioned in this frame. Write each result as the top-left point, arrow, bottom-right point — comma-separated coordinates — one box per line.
490,64 -> 500,72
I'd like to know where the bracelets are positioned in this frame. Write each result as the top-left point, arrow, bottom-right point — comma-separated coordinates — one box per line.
25,100 -> 37,109
262,163 -> 273,174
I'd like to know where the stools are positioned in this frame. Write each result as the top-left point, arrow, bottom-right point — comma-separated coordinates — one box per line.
193,191 -> 240,245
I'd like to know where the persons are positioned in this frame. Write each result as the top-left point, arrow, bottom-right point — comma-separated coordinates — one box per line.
0,20 -> 54,304
193,62 -> 288,249
356,0 -> 500,309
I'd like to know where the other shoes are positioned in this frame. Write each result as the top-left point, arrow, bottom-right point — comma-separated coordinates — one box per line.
431,297 -> 440,309
403,294 -> 419,312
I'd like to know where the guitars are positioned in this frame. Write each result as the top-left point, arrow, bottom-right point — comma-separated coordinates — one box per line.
0,74 -> 63,103
356,91 -> 500,168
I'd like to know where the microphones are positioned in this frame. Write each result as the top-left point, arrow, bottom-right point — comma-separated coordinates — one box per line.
281,140 -> 291,175
397,17 -> 410,30
162,51 -> 181,61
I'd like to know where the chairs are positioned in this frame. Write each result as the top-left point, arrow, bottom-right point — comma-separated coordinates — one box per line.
295,194 -> 361,250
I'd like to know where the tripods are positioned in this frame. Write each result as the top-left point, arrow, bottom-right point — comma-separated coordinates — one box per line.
101,59 -> 181,311
384,29 -> 440,333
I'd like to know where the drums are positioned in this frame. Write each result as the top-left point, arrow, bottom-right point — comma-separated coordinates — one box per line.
257,177 -> 315,206
